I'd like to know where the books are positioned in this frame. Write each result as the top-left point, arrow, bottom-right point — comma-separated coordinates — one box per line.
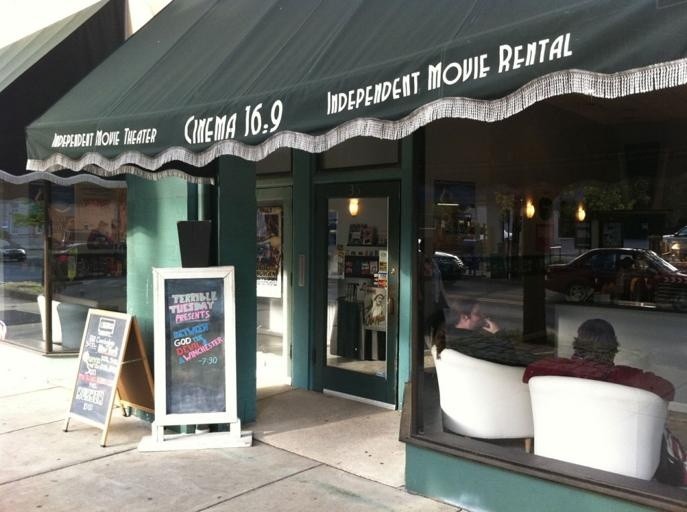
342,223 -> 387,300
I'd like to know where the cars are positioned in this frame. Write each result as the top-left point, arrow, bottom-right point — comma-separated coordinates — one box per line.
545,248 -> 687,312
52,228 -> 119,280
433,251 -> 465,285
0,239 -> 26,262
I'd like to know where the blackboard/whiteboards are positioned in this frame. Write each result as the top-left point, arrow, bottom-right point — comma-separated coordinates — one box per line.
69,308 -> 133,430
153,266 -> 238,425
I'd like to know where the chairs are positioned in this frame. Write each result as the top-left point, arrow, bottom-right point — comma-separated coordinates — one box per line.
433,347 -> 666,483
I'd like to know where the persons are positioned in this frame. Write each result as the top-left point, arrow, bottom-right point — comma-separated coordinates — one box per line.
435,298 -> 675,402
369,293 -> 387,311
62,215 -> 82,236
416,256 -> 449,352
86,220 -> 114,274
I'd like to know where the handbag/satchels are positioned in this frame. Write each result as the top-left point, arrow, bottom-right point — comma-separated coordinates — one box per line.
656,428 -> 686,487
